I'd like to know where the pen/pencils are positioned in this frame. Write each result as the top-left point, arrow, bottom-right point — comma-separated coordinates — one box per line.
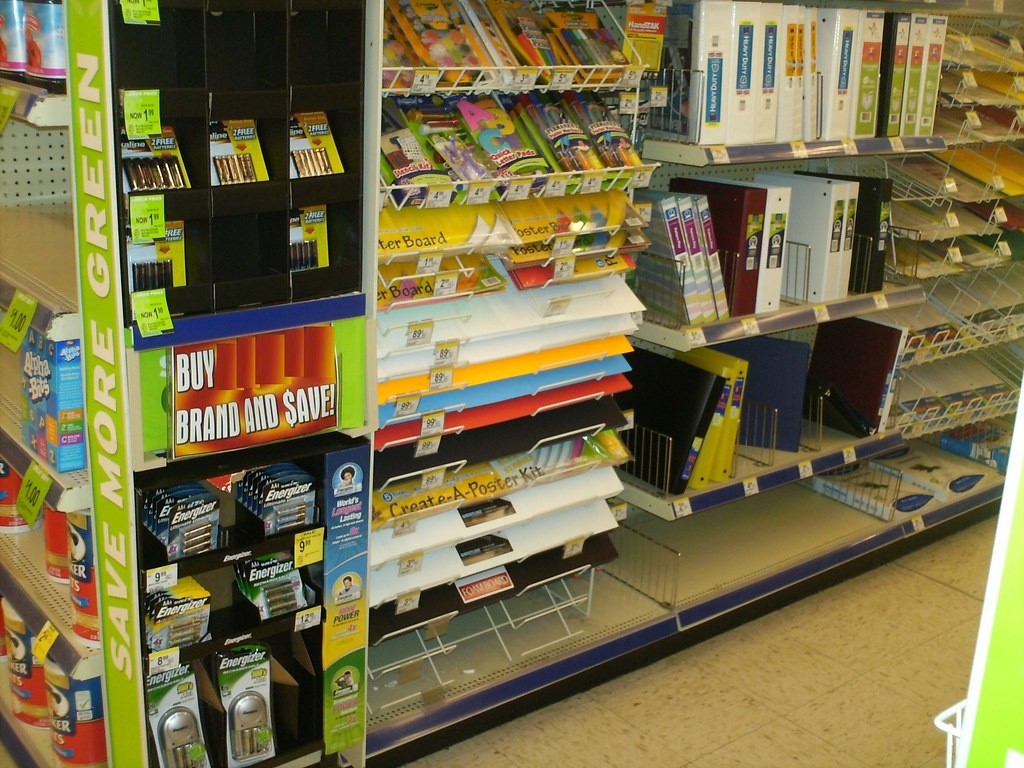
502,12 -> 645,180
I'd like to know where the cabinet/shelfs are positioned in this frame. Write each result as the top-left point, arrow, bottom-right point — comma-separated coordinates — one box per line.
0,2 -> 1022,767
